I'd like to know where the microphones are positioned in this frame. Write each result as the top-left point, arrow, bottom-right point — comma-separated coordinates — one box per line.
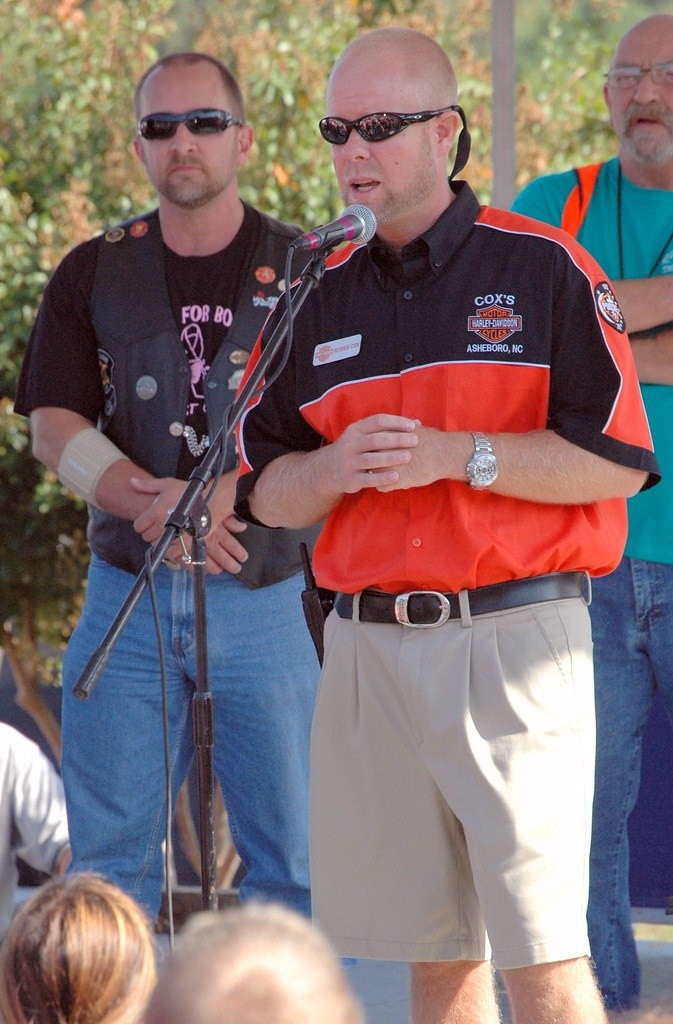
291,204 -> 377,251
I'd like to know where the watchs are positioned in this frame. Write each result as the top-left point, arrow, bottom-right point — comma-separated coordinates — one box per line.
467,431 -> 499,492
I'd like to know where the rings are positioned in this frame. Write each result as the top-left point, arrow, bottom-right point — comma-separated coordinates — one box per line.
368,469 -> 372,473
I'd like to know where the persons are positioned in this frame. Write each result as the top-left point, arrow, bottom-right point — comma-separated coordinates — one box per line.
234,13 -> 673,1024
0,52 -> 363,1024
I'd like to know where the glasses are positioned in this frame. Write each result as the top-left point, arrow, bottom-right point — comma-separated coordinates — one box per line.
319,104 -> 460,145
139,109 -> 245,139
605,63 -> 673,89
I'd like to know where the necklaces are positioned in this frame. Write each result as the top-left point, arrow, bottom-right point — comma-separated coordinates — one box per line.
618,156 -> 673,279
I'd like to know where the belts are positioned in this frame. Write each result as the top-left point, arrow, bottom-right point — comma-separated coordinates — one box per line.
335,571 -> 586,629
